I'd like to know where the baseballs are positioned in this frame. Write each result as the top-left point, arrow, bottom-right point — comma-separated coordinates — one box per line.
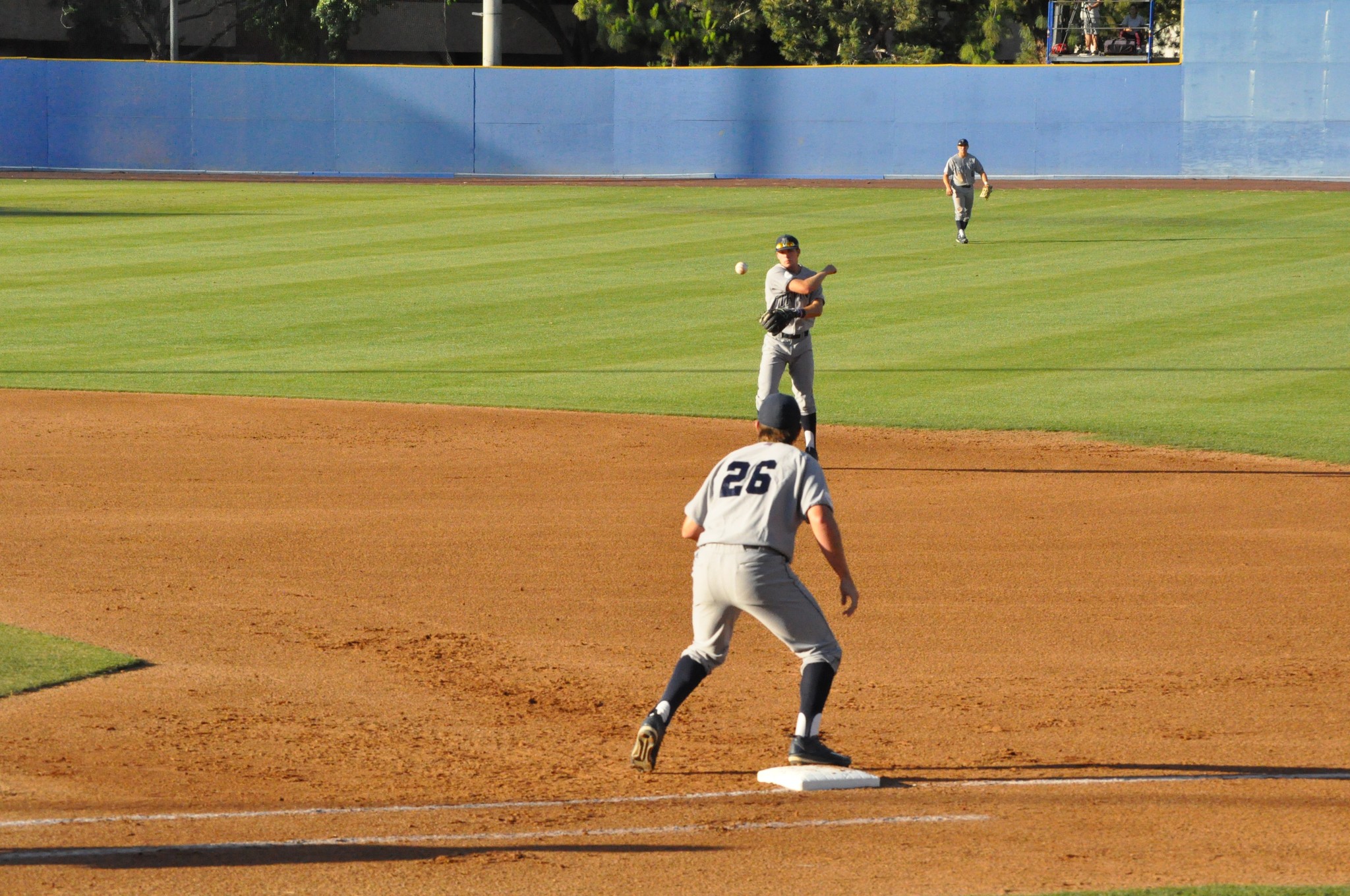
735,262 -> 748,275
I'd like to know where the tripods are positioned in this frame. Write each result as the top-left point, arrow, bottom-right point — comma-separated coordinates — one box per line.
1057,0 -> 1100,56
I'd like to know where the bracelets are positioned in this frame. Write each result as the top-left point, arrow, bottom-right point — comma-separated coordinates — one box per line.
984,181 -> 989,185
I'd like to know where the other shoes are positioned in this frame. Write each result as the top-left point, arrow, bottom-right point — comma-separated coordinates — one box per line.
1092,51 -> 1098,55
1079,50 -> 1091,55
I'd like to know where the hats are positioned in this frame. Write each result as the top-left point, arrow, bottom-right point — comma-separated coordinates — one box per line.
775,234 -> 798,251
958,139 -> 968,146
757,393 -> 801,430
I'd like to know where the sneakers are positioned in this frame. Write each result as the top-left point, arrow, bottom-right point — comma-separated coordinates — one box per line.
788,733 -> 851,768
805,446 -> 818,461
961,236 -> 968,243
956,233 -> 961,242
630,714 -> 664,773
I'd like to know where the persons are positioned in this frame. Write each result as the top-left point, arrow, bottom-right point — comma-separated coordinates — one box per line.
631,393 -> 860,772
1119,3 -> 1145,54
943,139 -> 991,243
1079,0 -> 1102,56
754,235 -> 837,462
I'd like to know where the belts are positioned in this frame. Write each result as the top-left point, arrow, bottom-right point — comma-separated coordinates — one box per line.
743,544 -> 758,550
782,331 -> 808,339
962,185 -> 970,188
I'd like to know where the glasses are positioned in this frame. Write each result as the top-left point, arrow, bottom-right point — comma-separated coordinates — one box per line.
958,142 -> 967,144
776,242 -> 797,249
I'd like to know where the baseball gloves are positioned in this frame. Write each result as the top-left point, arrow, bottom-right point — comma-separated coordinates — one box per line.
759,308 -> 806,336
980,184 -> 993,199
